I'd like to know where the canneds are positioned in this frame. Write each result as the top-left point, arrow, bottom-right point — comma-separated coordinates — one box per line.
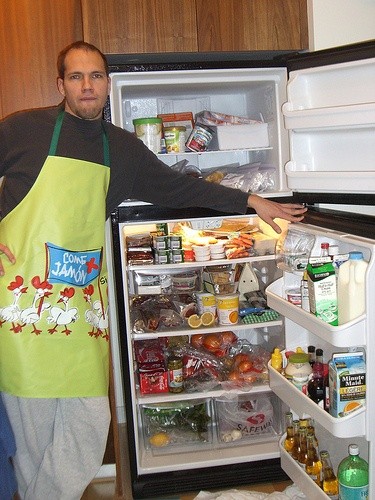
167,357 -> 185,393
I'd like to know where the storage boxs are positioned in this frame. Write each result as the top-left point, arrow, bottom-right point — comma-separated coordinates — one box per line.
215,395 -> 283,444
211,121 -> 269,150
141,400 -> 213,454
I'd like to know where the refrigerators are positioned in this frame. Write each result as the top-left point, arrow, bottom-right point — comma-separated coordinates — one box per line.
104,39 -> 375,500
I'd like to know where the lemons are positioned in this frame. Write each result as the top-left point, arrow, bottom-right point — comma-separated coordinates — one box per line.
149,432 -> 170,446
188,312 -> 215,328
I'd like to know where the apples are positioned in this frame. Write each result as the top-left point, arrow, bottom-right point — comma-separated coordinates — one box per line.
190,332 -> 258,386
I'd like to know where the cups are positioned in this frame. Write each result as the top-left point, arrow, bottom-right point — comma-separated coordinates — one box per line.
186,121 -> 215,152
162,126 -> 186,154
133,117 -> 163,154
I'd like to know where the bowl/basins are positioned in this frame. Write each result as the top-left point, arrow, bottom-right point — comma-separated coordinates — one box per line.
172,273 -> 197,290
285,288 -> 301,307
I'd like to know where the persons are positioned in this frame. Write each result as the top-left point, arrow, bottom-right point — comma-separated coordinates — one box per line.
0,40 -> 307,500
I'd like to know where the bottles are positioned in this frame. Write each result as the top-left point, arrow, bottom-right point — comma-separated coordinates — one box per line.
320,450 -> 338,500
292,373 -> 308,397
324,374 -> 329,413
307,349 -> 324,405
285,427 -> 294,454
291,418 -> 308,470
271,348 -> 283,374
306,426 -> 323,489
321,243 -> 329,257
284,412 -> 293,427
337,251 -> 369,326
337,444 -> 369,500
285,353 -> 312,383
307,345 -> 315,367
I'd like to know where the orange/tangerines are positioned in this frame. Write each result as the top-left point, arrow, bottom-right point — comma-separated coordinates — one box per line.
344,401 -> 360,412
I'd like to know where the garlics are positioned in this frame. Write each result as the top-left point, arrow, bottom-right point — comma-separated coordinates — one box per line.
223,429 -> 243,443
